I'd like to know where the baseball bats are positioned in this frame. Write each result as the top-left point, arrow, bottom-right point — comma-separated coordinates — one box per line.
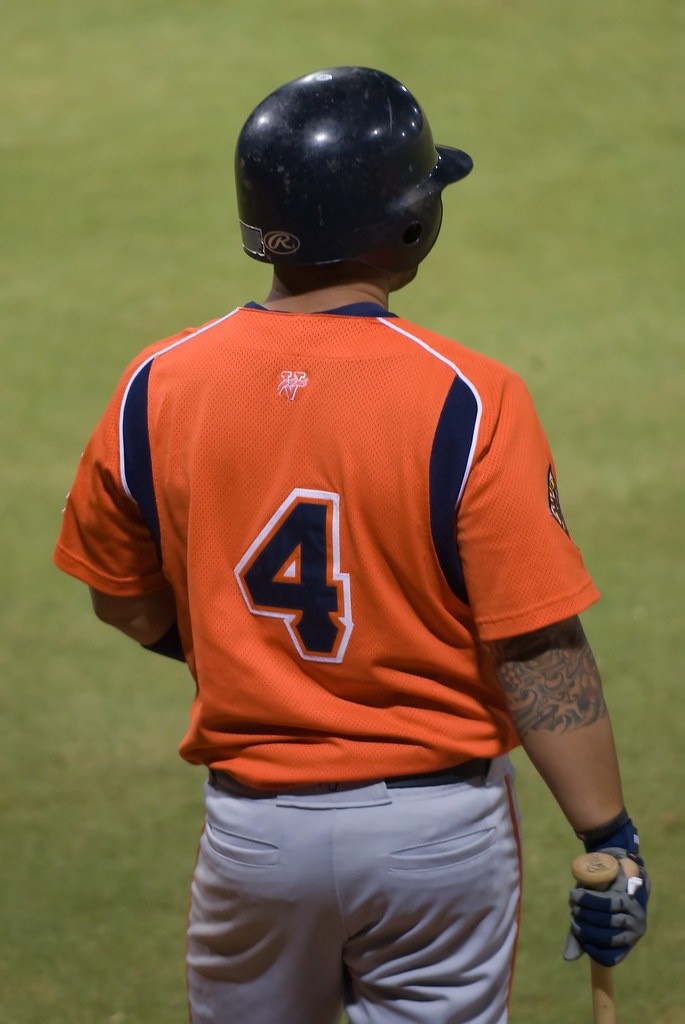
571,854 -> 621,1022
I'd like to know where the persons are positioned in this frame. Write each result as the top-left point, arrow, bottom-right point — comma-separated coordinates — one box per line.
49,66 -> 652,1024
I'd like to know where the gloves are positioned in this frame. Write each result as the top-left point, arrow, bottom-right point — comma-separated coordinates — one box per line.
561,806 -> 651,968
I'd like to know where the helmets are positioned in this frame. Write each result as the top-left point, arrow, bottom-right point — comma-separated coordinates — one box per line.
233,65 -> 474,267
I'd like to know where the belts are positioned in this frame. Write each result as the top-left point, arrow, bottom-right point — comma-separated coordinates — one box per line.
207,756 -> 493,800
